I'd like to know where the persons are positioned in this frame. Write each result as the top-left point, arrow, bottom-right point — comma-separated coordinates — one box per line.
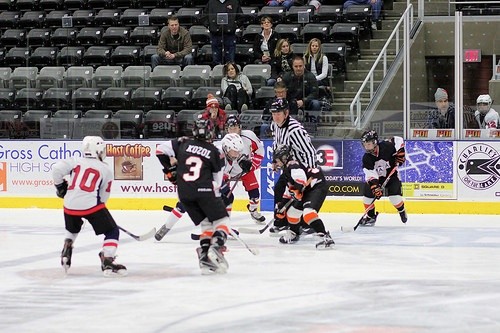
254,16 -> 281,64
53,136 -> 128,271
271,144 -> 336,248
267,39 -> 299,86
156,115 -> 231,270
473,94 -> 500,128
201,0 -> 245,68
282,56 -> 320,111
343,0 -> 382,30
221,61 -> 253,113
224,116 -> 266,222
151,15 -> 194,70
155,133 -> 252,241
262,82 -> 298,121
270,99 -> 317,232
358,130 -> 408,225
427,87 -> 469,129
266,0 -> 322,16
192,95 -> 227,139
302,39 -> 330,96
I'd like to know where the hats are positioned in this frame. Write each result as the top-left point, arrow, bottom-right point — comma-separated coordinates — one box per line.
206,94 -> 219,108
435,88 -> 448,100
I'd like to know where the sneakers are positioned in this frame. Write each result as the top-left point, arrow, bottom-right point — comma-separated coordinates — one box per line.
196,229 -> 228,275
228,229 -> 239,239
247,204 -> 266,224
359,212 -> 378,225
269,225 -> 335,249
400,211 -> 407,223
155,225 -> 170,241
98,250 -> 127,277
61,238 -> 73,274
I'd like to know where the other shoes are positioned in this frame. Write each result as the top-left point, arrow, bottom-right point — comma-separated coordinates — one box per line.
225,103 -> 248,111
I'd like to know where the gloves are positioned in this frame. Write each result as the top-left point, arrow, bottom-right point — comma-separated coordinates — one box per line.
250,153 -> 264,171
221,187 -> 234,204
161,165 -> 178,185
370,180 -> 383,199
276,208 -> 286,218
54,179 -> 68,198
289,188 -> 303,200
393,148 -> 405,166
237,154 -> 250,172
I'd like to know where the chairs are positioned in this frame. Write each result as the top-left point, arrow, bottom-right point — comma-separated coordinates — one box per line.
0,0 -> 371,139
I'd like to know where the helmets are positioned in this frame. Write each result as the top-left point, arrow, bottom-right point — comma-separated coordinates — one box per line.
270,97 -> 289,113
193,118 -> 214,140
220,133 -> 243,161
225,115 -> 242,130
361,131 -> 378,145
81,136 -> 106,161
476,94 -> 491,113
272,144 -> 290,159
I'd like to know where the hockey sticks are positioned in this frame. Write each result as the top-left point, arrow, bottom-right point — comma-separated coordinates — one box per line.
191,170 -> 246,240
341,162 -> 399,232
163,154 -> 248,212
220,172 -> 242,190
230,229 -> 259,257
238,178 -> 312,234
119,225 -> 156,242
